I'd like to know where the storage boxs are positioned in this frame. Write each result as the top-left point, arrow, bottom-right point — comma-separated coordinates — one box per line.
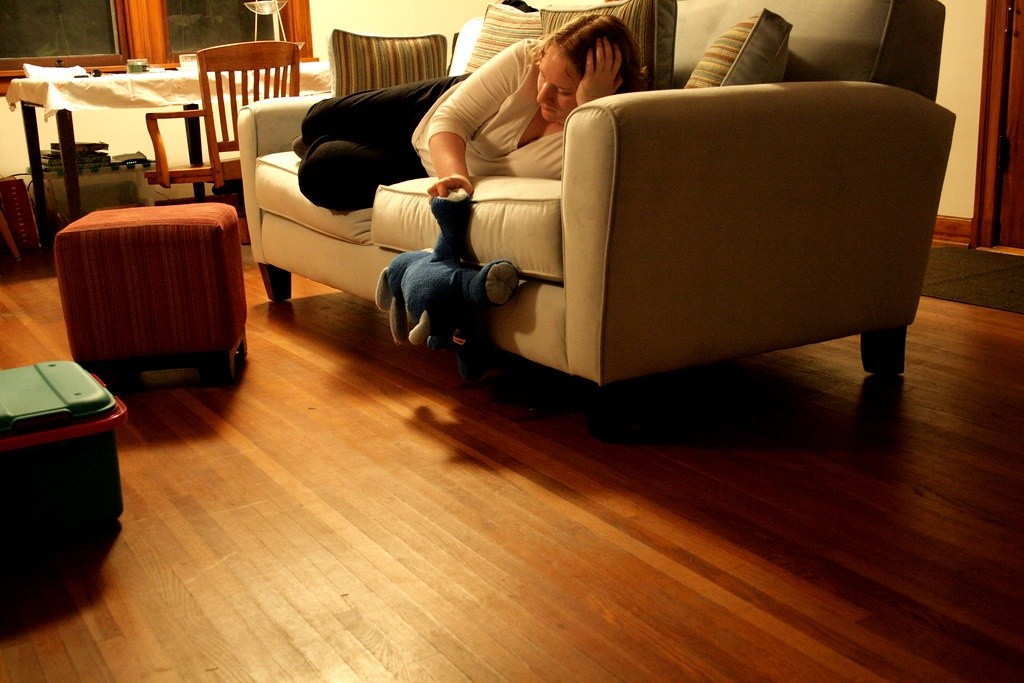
0,358 -> 128,580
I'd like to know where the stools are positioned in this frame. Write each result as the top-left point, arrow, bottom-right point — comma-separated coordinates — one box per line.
52,201 -> 248,395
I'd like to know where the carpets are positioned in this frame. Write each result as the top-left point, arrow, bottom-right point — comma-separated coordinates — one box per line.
921,246 -> 1024,315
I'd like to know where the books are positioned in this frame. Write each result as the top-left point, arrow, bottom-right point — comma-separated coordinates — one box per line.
28,142 -> 112,179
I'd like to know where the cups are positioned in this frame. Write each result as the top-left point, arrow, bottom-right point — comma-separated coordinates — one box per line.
179,53 -> 197,67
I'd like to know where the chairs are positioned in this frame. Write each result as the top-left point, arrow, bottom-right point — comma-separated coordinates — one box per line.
0,211 -> 20,259
143,41 -> 300,244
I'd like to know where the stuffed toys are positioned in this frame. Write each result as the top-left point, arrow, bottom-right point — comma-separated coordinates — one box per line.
374,187 -> 519,383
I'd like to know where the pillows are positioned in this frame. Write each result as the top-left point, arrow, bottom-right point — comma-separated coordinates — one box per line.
539,0 -> 678,93
461,4 -> 543,75
683,7 -> 794,89
327,28 -> 448,96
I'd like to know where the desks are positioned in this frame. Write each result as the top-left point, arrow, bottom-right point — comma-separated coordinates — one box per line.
4,61 -> 332,253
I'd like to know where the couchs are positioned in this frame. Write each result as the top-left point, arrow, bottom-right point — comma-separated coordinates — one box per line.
237,0 -> 957,445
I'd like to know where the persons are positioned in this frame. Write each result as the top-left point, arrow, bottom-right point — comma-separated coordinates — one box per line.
293,15 -> 648,210
